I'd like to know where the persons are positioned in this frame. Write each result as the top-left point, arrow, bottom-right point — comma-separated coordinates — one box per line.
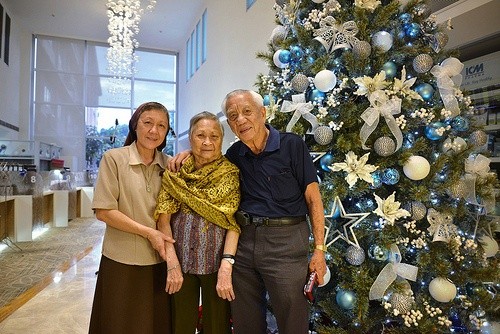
87,101 -> 174,334
153,111 -> 243,334
166,87 -> 328,334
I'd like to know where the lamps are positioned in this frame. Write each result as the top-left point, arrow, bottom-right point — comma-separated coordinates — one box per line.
104,0 -> 157,96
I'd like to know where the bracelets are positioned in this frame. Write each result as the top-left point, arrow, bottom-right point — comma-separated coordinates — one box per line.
166,262 -> 182,273
314,244 -> 328,254
222,254 -> 236,265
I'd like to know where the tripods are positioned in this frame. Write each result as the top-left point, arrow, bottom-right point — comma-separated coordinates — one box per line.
0,183 -> 22,253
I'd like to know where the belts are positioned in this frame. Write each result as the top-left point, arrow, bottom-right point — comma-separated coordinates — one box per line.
249,215 -> 307,226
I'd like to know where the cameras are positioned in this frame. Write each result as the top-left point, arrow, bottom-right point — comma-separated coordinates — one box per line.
304,271 -> 319,302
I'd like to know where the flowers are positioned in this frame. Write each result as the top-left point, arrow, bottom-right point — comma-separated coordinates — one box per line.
258,0 -> 483,329
